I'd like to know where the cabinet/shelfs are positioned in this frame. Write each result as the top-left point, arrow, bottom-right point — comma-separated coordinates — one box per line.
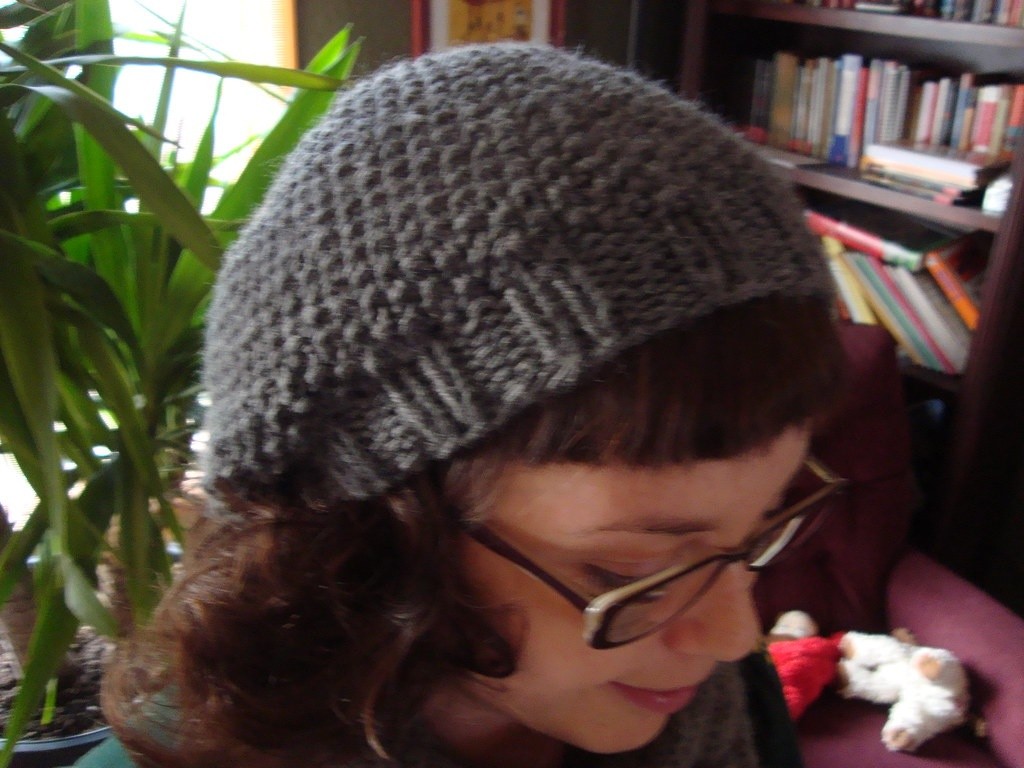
677,0 -> 1024,561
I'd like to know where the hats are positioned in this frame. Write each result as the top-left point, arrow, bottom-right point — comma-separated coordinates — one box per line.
182,42 -> 837,522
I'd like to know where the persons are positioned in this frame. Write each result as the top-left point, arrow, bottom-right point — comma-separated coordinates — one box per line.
68,40 -> 849,768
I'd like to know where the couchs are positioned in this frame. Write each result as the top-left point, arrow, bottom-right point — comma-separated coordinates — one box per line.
754,321 -> 1024,768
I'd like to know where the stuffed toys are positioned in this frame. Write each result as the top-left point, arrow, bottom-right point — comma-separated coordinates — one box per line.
823,619 -> 983,751
762,605 -> 844,727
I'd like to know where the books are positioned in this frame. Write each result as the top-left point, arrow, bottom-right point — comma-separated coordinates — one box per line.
650,0 -> 1024,382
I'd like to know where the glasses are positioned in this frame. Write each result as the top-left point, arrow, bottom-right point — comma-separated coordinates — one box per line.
447,454 -> 846,650
